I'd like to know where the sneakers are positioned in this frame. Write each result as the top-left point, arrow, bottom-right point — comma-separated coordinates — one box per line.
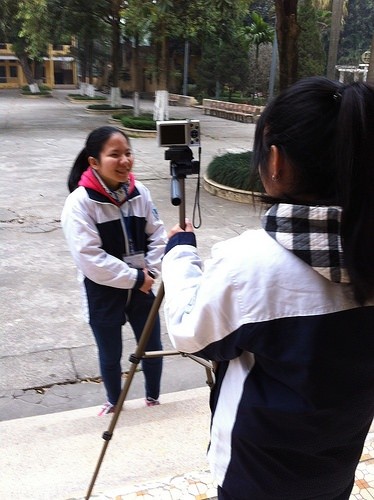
98,401 -> 121,415
146,397 -> 159,407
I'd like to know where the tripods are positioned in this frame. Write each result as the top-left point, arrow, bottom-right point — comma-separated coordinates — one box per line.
83,147 -> 218,500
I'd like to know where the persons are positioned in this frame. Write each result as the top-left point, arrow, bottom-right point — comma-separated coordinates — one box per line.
160,75 -> 374,499
61,124 -> 169,418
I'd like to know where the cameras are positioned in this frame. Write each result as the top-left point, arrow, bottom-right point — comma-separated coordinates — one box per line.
155,118 -> 201,147
